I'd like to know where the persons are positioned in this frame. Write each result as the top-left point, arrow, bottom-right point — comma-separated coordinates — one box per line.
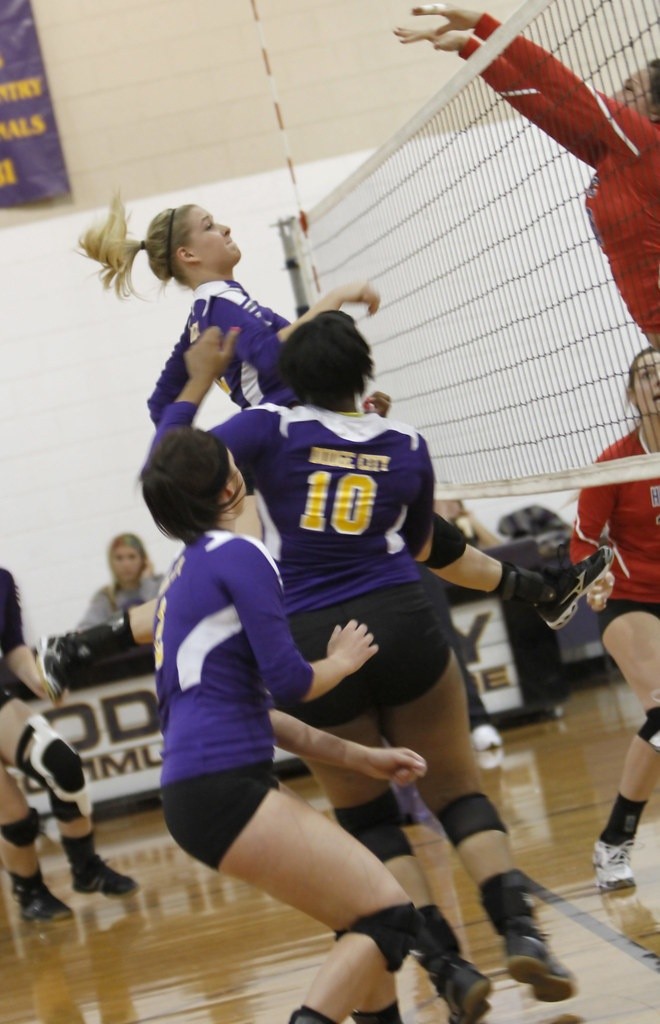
139,427 -> 426,1024
0,566 -> 141,922
79,190 -> 614,630
433,480 -> 500,550
24,918 -> 146,1024
147,310 -> 578,1024
569,346 -> 660,889
80,533 -> 164,631
391,4 -> 660,353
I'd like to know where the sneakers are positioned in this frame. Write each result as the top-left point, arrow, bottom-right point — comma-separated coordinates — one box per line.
35,632 -> 73,706
21,917 -> 76,969
19,886 -> 73,923
591,834 -> 636,890
503,934 -> 578,1002
532,545 -> 614,631
601,885 -> 652,931
436,956 -> 492,1024
477,747 -> 504,770
472,723 -> 503,751
87,915 -> 145,960
70,854 -> 140,899
